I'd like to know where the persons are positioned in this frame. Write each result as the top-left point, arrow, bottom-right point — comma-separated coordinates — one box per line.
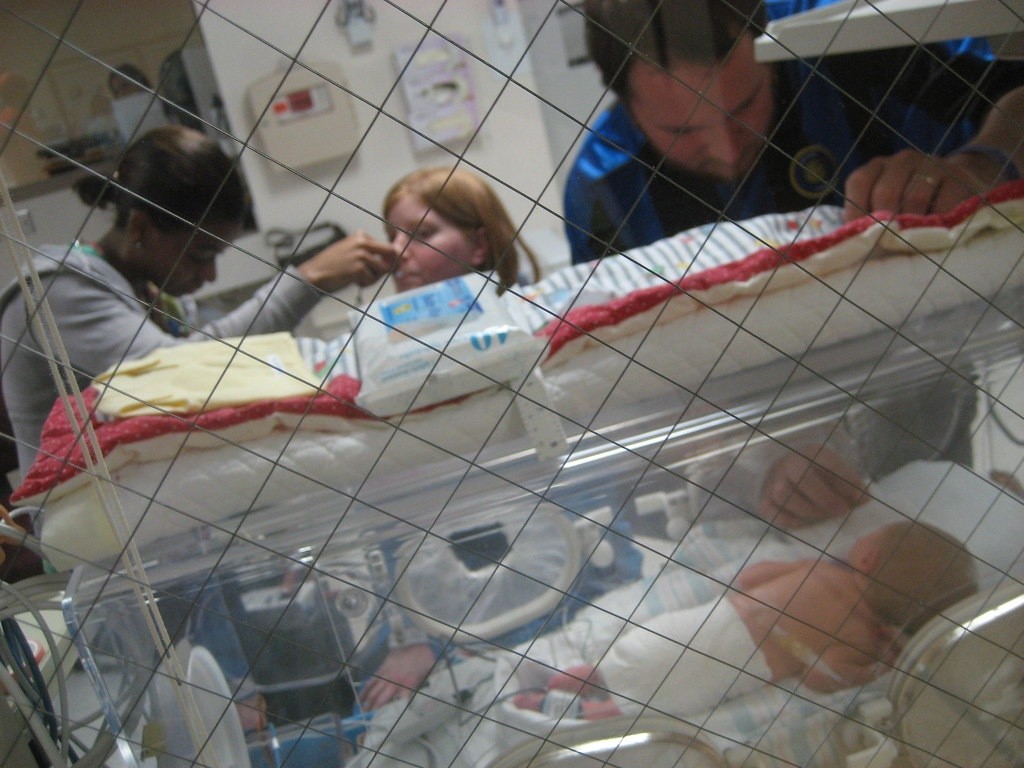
0,124 -> 411,733
563,0 -> 1024,538
354,170 -> 585,707
1,72 -> 46,187
108,64 -> 180,126
514,522 -> 978,720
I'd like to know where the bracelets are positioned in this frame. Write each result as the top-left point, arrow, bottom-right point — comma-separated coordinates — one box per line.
948,144 -> 1022,180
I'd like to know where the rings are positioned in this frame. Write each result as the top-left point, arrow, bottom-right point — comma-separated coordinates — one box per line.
913,174 -> 939,187
